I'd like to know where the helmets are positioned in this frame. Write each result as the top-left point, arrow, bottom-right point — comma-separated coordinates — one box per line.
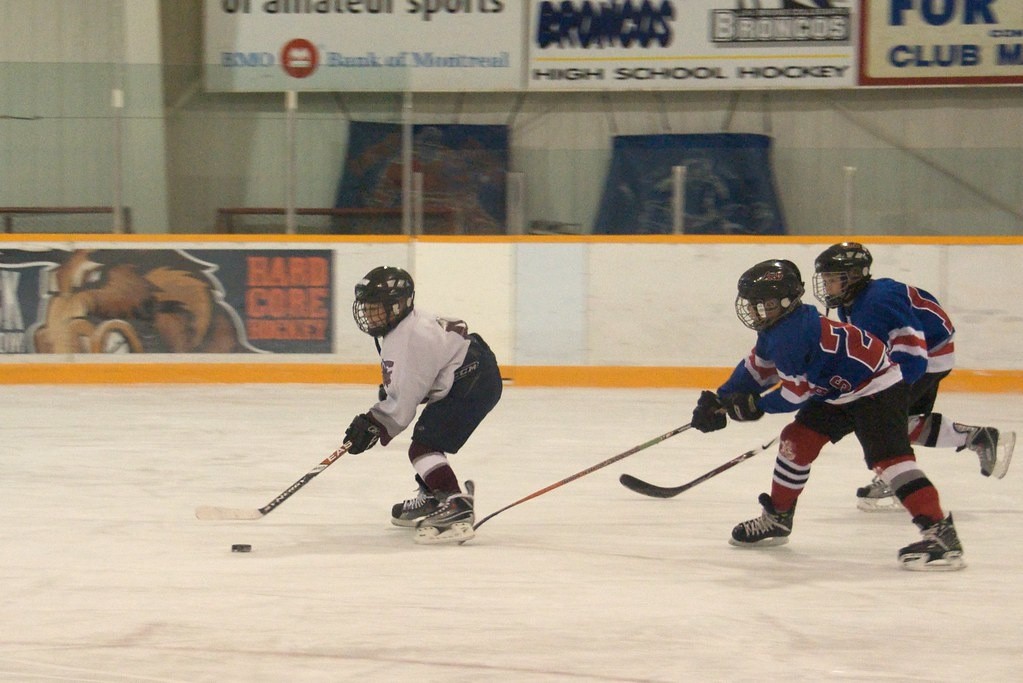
352,266 -> 413,336
811,241 -> 872,308
734,257 -> 805,329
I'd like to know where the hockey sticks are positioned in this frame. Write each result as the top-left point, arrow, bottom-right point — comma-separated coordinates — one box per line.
194,437 -> 355,522
457,406 -> 730,549
618,433 -> 781,501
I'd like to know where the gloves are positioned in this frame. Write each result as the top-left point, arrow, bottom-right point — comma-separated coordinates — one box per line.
343,413 -> 381,454
726,390 -> 765,421
691,390 -> 726,432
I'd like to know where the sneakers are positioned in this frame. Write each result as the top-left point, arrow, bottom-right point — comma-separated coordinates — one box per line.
390,487 -> 443,527
731,492 -> 796,546
414,480 -> 474,544
953,422 -> 1015,479
897,511 -> 965,571
857,479 -> 907,509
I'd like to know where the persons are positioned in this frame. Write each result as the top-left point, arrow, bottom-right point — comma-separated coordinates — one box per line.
342,266 -> 503,543
691,259 -> 964,563
812,241 -> 998,501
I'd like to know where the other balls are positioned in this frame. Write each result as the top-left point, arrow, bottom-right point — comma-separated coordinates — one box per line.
231,543 -> 252,554
